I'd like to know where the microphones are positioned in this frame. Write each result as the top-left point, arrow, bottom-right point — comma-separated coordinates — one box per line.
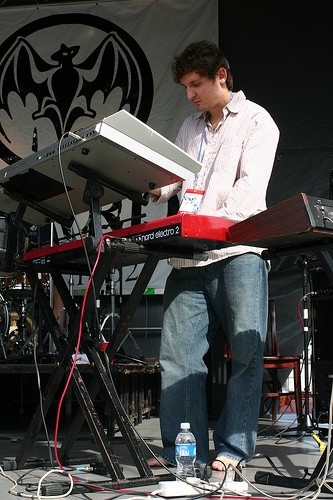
32,128 -> 37,151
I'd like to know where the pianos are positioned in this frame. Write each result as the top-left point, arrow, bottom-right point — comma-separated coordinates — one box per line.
0,109 -> 204,228
0,213 -> 243,497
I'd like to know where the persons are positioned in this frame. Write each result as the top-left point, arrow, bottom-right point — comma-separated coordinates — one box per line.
146,39 -> 278,470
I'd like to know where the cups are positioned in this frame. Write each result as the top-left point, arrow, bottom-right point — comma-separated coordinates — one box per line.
98,341 -> 114,365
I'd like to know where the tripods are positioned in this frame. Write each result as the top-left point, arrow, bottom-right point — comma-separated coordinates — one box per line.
97,268 -> 145,363
2,300 -> 44,358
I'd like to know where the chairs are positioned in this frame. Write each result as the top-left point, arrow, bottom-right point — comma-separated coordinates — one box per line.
225,301 -> 303,425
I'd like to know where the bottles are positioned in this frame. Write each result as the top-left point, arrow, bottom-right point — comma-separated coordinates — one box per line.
175,422 -> 196,482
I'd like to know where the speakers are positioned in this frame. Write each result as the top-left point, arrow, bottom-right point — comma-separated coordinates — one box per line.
308,267 -> 333,424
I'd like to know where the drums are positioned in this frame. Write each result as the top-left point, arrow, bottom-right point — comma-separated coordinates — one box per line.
0,217 -> 28,278
0,293 -> 11,344
0,272 -> 50,297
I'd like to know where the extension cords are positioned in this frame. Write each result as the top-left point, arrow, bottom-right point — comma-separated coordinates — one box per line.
158,481 -> 248,497
